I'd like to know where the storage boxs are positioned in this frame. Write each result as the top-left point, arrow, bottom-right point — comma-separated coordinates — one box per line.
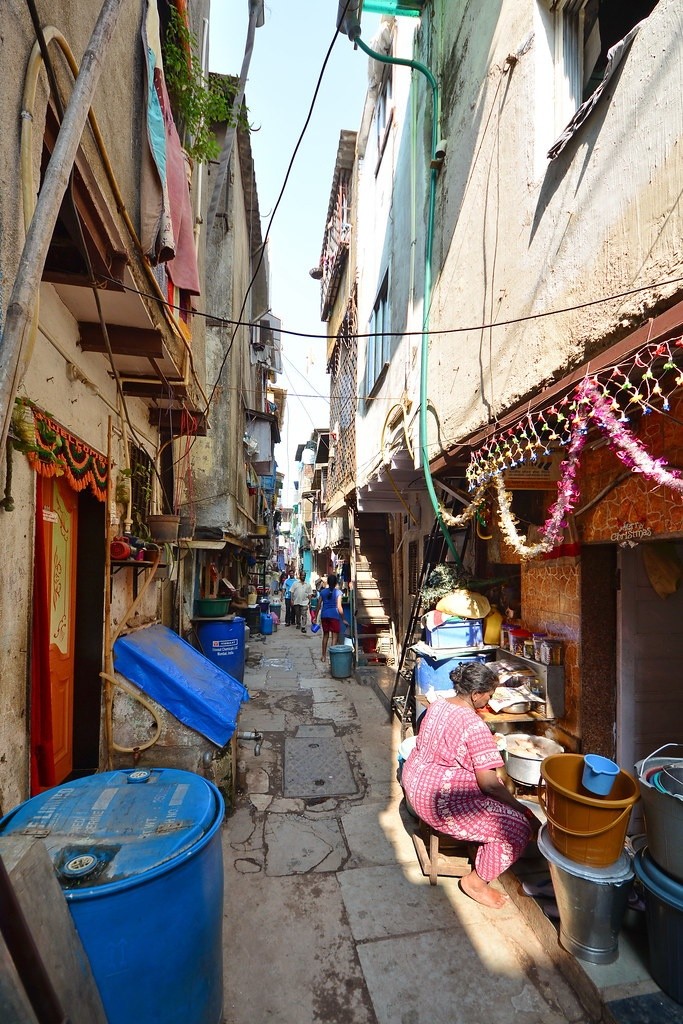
425,619 -> 484,648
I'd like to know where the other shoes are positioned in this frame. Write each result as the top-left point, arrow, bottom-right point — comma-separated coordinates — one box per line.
290,621 -> 295,625
286,622 -> 289,626
296,623 -> 301,629
301,628 -> 306,633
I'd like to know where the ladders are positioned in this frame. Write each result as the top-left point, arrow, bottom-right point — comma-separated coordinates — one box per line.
389,485 -> 457,742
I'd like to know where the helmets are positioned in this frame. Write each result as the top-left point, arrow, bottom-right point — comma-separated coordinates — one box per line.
282,570 -> 285,573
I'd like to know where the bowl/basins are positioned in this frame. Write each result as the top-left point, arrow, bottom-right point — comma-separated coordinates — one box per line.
194,598 -> 232,617
501,702 -> 531,714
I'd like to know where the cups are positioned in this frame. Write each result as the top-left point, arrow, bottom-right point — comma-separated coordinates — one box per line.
311,624 -> 320,633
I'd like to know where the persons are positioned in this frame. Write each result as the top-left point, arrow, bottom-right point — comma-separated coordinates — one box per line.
279,569 -> 351,663
401,661 -> 534,909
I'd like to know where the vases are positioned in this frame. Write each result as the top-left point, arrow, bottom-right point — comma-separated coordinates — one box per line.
256,525 -> 268,535
249,487 -> 256,495
146,515 -> 196,543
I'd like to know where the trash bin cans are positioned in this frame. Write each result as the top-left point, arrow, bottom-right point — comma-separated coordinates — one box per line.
269,602 -> 281,620
329,644 -> 353,679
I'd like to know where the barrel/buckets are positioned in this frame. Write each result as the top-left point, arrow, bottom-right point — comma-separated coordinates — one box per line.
482,604 -> 503,644
259,613 -> 272,635
196,617 -> 245,685
415,651 -> 491,735
537,743 -> 683,1006
0,768 -> 225,1024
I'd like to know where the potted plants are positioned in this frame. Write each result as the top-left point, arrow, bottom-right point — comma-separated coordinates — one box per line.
141,523 -> 177,581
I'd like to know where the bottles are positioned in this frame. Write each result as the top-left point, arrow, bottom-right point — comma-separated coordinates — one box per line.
500,624 -> 563,666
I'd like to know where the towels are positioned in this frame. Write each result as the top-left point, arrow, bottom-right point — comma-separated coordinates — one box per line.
420,609 -> 453,632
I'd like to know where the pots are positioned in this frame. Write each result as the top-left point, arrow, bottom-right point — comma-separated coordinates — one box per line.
501,734 -> 564,786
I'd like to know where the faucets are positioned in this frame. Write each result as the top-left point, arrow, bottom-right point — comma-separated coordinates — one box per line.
237,728 -> 265,757
